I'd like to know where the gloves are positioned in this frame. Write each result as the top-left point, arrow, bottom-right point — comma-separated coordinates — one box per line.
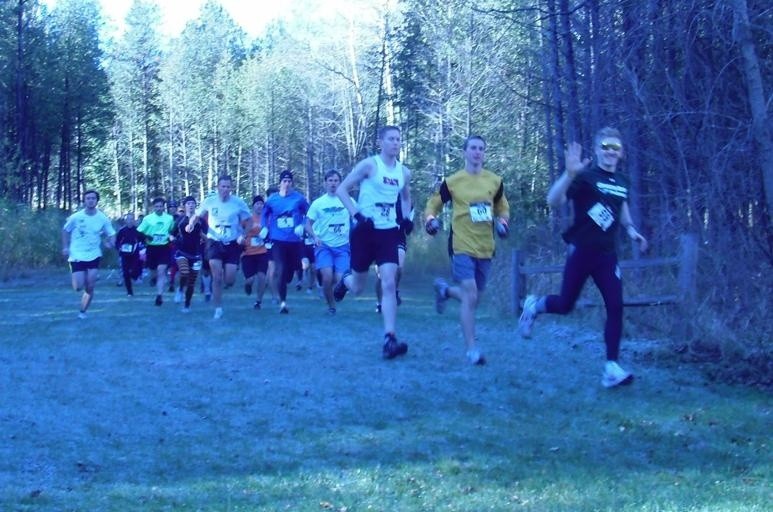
294,225 -> 304,237
402,218 -> 414,236
425,215 -> 439,235
259,228 -> 269,239
354,212 -> 374,233
497,218 -> 509,238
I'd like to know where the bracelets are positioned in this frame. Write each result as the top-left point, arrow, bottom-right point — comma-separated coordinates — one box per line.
624,223 -> 639,231
425,215 -> 435,222
500,218 -> 509,230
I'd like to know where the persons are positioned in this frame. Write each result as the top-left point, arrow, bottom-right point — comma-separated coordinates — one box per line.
517,127 -> 648,388
62,190 -> 118,316
423,136 -> 510,364
333,125 -> 408,359
111,170 -> 415,318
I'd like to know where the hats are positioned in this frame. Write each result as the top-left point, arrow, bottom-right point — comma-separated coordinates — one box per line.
252,195 -> 264,205
168,196 -> 196,212
281,170 -> 293,182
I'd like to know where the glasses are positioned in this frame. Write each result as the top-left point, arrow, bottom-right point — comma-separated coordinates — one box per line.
600,142 -> 622,151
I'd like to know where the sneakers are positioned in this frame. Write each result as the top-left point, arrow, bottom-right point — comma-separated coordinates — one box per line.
327,308 -> 335,315
432,277 -> 448,312
396,291 -> 401,305
333,269 -> 353,302
214,306 -> 223,319
150,278 -> 211,313
244,283 -> 289,313
466,349 -> 485,364
286,270 -> 294,283
382,340 -> 408,358
79,312 -> 87,319
375,302 -> 381,313
601,362 -> 634,387
519,294 -> 541,341
296,281 -> 327,297
